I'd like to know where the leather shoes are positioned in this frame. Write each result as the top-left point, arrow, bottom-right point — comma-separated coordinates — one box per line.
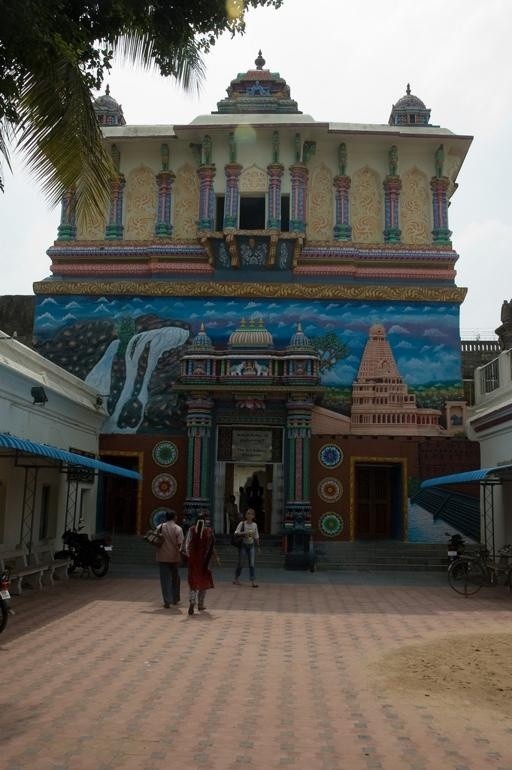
164,598 -> 206,615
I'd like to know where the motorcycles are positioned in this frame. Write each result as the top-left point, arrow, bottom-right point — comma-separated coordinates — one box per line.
55,519 -> 114,578
444,533 -> 466,576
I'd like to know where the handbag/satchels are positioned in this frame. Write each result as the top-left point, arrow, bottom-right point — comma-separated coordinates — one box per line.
143,526 -> 165,547
229,524 -> 245,547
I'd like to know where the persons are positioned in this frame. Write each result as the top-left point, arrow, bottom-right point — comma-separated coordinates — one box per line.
181,513 -> 222,616
239,486 -> 249,514
250,81 -> 263,96
152,511 -> 186,607
227,496 -> 238,534
231,509 -> 261,587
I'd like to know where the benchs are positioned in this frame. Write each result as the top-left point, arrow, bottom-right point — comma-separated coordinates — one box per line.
0,545 -> 71,597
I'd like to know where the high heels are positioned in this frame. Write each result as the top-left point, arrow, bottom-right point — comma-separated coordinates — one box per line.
248,578 -> 259,588
233,577 -> 239,585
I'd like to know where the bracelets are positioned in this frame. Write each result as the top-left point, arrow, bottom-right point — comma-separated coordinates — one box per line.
216,558 -> 220,562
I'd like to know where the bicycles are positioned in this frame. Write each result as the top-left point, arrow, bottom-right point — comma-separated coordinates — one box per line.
449,546 -> 512,598
